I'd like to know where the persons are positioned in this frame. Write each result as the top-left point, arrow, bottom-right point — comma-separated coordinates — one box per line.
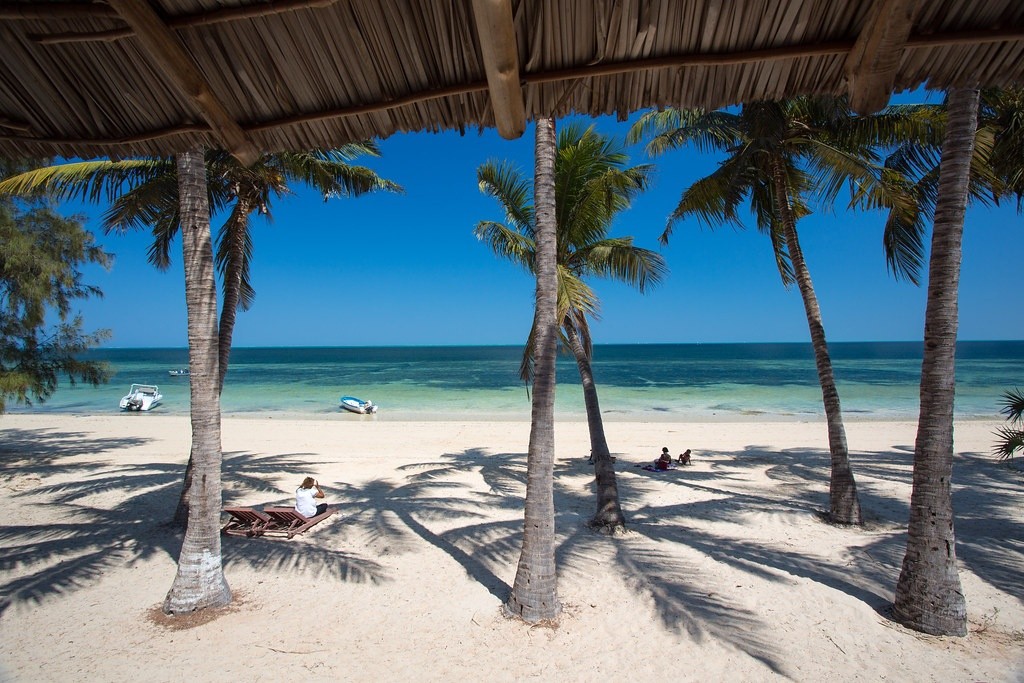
655,447 -> 671,471
675,449 -> 691,466
295,476 -> 328,517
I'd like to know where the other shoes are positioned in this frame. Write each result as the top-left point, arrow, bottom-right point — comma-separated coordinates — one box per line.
675,460 -> 679,463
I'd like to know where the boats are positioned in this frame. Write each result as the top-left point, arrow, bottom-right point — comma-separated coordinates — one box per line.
340,394 -> 378,414
168,370 -> 190,377
120,380 -> 164,412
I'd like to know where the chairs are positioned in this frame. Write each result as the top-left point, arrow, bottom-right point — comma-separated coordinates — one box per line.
221,506 -> 331,540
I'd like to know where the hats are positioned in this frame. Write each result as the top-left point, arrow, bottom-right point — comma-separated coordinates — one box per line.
662,447 -> 668,452
